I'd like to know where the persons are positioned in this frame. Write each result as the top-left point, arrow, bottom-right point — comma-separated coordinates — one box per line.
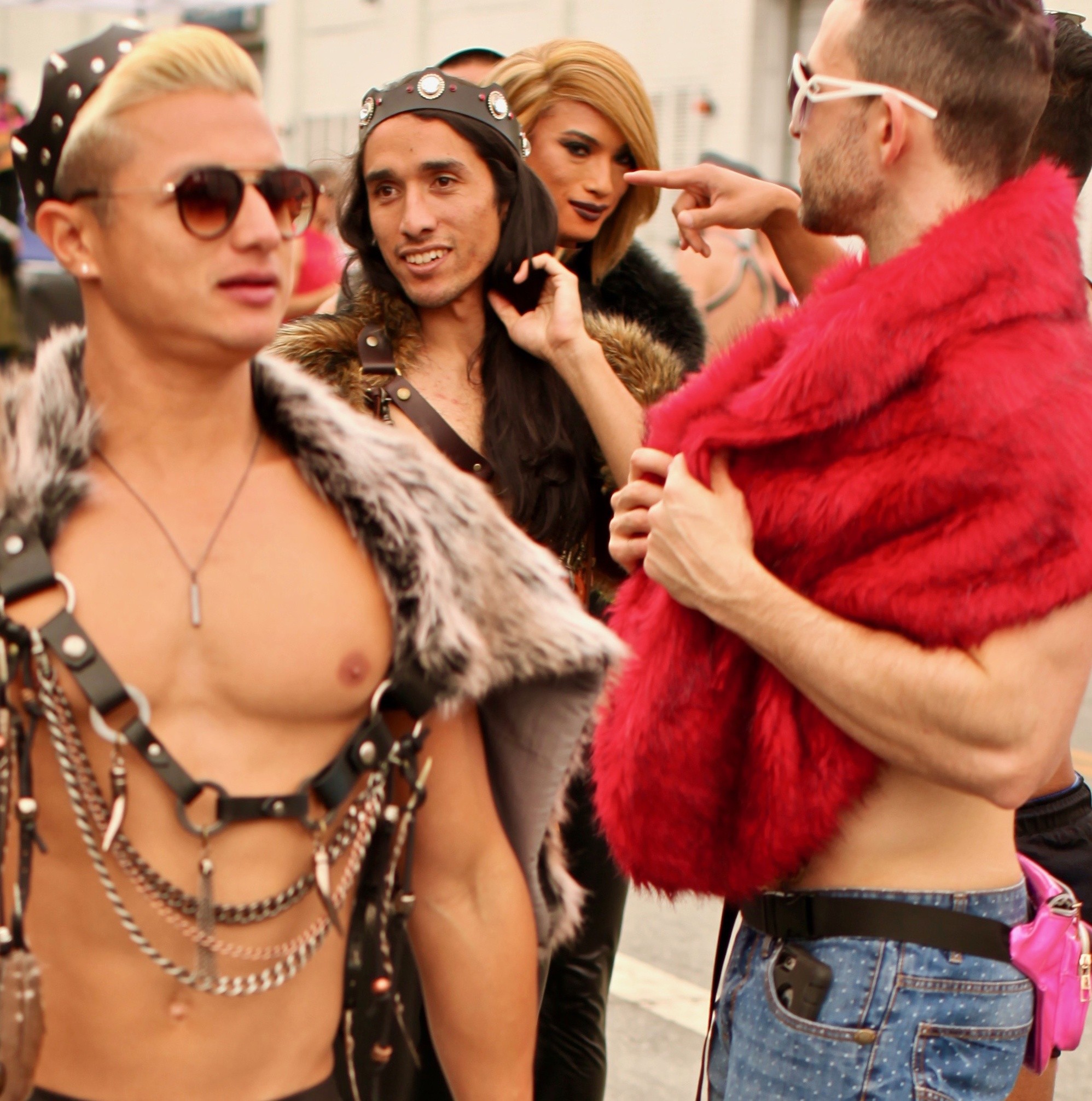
1,25 -> 620,1101
274,28 -> 702,1101
287,164 -> 354,318
602,2 -> 1092,1100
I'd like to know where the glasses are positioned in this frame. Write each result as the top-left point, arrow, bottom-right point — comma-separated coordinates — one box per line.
68,162 -> 324,239
779,50 -> 938,136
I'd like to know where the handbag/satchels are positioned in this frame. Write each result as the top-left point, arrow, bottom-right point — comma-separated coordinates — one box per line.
1010,853 -> 1091,1076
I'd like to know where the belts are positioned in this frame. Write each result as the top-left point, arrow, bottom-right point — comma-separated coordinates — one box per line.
737,882 -> 1011,968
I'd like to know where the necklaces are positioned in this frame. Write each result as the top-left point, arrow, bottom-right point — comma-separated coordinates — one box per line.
83,435 -> 266,629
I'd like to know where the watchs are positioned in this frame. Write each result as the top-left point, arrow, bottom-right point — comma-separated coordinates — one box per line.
654,149 -> 776,372
